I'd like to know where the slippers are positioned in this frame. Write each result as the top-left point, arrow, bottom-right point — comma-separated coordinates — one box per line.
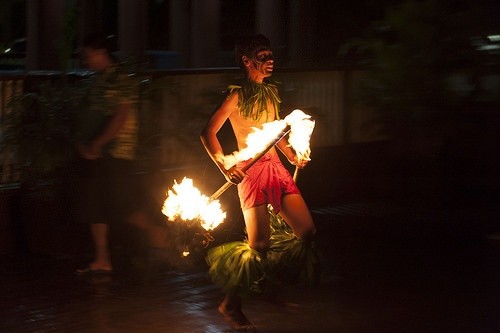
71,262 -> 115,275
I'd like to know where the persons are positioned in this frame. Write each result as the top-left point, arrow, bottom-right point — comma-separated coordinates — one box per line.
73,36 -> 172,275
199,32 -> 316,332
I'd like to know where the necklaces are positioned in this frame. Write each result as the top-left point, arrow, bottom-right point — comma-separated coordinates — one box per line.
210,73 -> 283,121
